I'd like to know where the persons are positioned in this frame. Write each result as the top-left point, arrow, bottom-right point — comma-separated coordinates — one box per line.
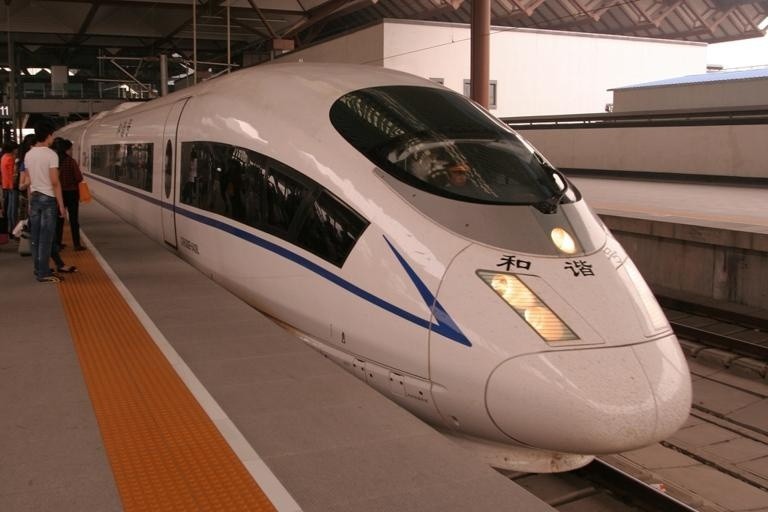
410,132 -> 470,186
112,144 -> 148,184
0,120 -> 87,282
182,149 -> 245,220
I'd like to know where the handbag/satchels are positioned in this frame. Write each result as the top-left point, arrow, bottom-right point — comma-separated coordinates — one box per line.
19,231 -> 33,256
80,182 -> 91,203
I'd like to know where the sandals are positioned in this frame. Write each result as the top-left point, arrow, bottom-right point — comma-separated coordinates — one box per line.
59,264 -> 78,273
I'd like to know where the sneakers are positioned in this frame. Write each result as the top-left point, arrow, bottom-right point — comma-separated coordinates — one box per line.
33,268 -> 63,282
75,245 -> 87,251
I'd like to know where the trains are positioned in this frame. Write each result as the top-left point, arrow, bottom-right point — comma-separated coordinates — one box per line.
52,62 -> 692,473
